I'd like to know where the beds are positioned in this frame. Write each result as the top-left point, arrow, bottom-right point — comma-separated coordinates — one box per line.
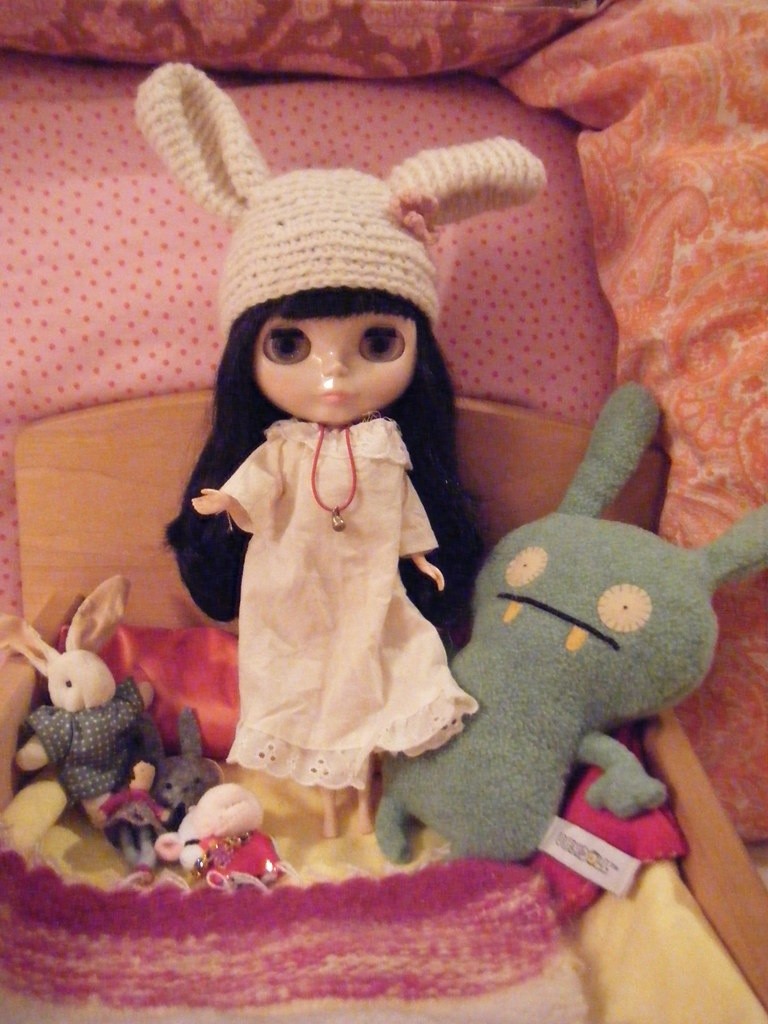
0,389 -> 768,1024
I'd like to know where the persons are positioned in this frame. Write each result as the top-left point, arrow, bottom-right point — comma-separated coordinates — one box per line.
133,60 -> 549,845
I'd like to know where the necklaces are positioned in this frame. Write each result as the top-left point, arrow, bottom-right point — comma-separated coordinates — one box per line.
311,424 -> 358,531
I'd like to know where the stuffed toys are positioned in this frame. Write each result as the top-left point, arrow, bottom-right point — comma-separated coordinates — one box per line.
1,573 -> 291,890
373,380 -> 768,865
